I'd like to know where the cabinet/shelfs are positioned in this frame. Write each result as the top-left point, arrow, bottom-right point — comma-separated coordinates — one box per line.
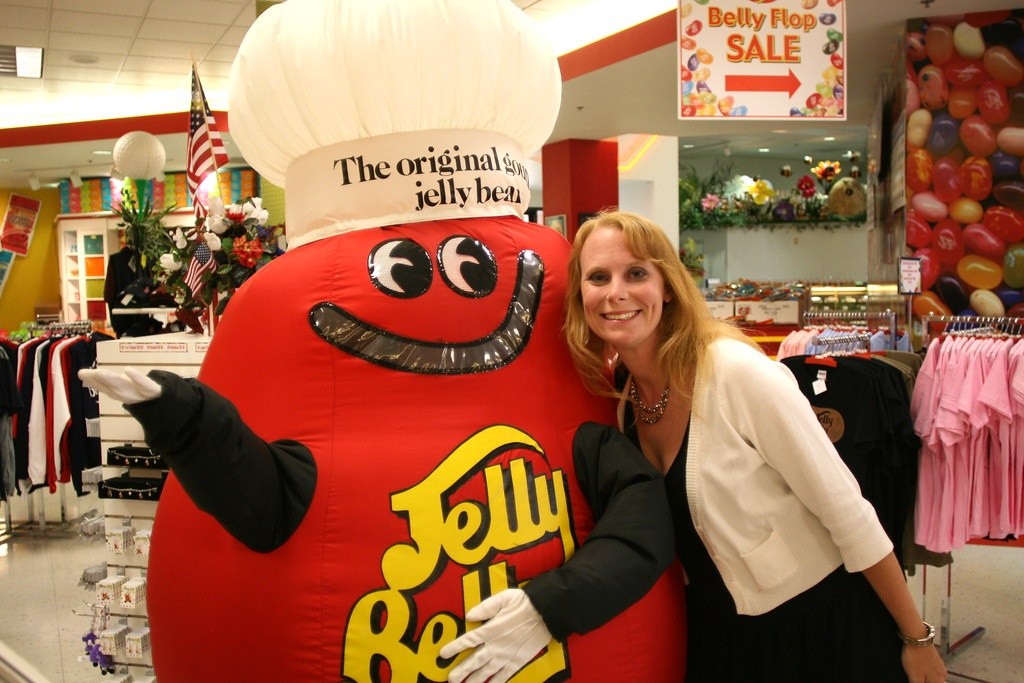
56,205 -> 233,328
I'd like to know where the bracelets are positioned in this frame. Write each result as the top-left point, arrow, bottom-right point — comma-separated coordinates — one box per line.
899,622 -> 936,647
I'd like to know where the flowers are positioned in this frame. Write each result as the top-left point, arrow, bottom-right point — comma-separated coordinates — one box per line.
158,195 -> 285,315
680,144 -> 865,234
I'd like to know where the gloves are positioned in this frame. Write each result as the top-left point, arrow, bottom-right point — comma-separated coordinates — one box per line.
440,588 -> 552,683
76,366 -> 161,406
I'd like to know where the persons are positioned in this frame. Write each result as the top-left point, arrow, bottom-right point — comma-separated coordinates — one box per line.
565,211 -> 946,683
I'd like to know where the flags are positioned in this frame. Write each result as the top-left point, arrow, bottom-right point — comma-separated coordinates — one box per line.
183,59 -> 230,225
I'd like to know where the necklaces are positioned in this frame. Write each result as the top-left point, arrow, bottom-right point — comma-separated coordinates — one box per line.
629,377 -> 670,423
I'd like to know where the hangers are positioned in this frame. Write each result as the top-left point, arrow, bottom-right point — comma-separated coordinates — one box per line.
30,319 -> 93,339
801,309 -> 1024,370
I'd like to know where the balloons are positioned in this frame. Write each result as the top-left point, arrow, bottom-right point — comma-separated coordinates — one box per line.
906,8 -> 1023,331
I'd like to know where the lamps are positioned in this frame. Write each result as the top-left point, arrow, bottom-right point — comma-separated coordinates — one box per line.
26,165 -> 124,191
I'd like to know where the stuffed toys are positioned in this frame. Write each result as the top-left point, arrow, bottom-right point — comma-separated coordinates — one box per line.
76,0 -> 689,683
